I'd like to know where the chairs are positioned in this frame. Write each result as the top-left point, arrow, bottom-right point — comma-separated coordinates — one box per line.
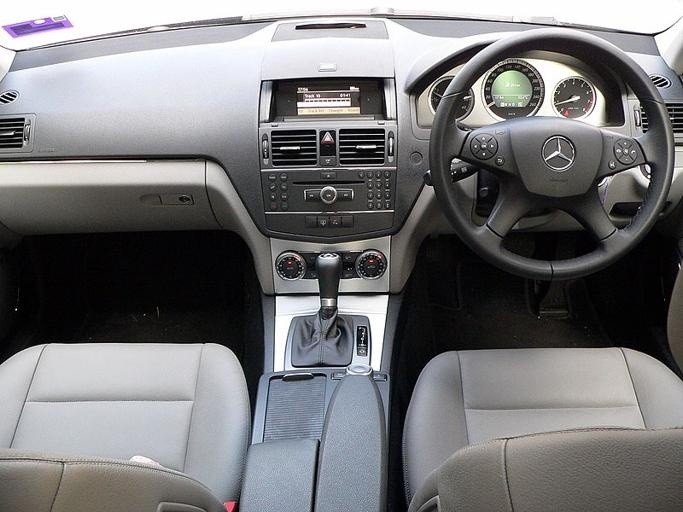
0,337 -> 252,511
399,341 -> 683,510
